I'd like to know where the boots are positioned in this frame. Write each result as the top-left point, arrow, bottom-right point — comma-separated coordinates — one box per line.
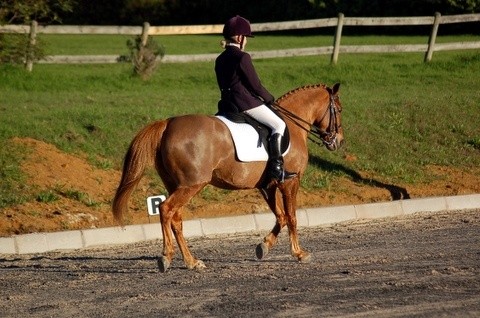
269,133 -> 297,182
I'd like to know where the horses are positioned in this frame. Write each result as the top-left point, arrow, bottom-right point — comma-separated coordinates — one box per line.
111,82 -> 344,271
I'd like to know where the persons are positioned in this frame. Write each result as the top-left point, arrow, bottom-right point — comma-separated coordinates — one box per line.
215,15 -> 298,180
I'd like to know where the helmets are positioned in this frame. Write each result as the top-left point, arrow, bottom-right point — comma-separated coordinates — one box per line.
223,15 -> 254,40
